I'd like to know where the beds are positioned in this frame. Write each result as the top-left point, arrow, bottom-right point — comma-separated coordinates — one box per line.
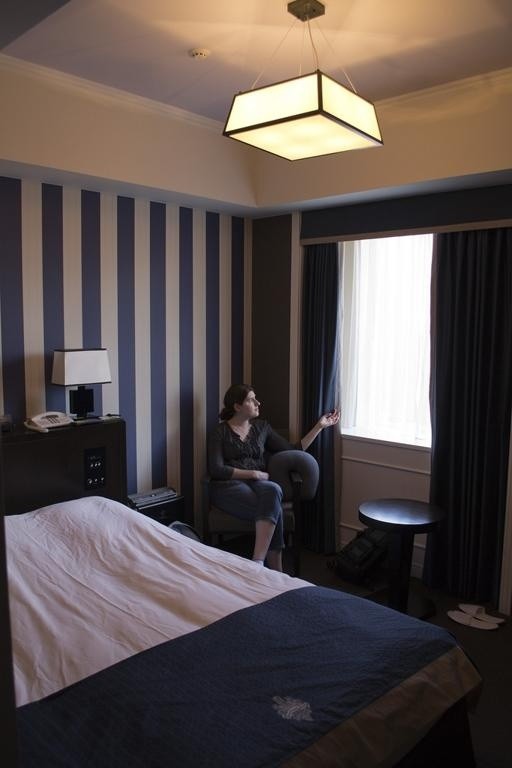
5,492 -> 467,768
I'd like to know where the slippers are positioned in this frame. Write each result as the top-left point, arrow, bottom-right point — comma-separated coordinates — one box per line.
446,601 -> 504,630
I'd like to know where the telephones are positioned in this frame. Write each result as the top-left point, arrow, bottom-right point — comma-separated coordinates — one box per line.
30,410 -> 74,429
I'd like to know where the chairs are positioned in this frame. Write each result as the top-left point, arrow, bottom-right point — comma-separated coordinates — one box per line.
200,428 -> 303,577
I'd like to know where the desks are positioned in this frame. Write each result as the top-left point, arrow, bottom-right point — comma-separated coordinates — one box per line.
357,498 -> 447,619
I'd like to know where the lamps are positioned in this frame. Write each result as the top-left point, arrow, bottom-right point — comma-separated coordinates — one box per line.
51,347 -> 112,421
221,1 -> 386,164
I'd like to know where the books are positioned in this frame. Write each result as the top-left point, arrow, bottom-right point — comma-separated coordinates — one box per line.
127,485 -> 175,504
136,493 -> 178,507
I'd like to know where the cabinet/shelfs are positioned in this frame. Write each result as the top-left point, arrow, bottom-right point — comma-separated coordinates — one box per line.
1,416 -> 128,515
134,496 -> 184,527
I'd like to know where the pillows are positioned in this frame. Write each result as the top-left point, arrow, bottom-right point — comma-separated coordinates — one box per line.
267,448 -> 321,501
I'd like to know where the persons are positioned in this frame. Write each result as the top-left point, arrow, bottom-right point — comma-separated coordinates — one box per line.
206,380 -> 341,574
